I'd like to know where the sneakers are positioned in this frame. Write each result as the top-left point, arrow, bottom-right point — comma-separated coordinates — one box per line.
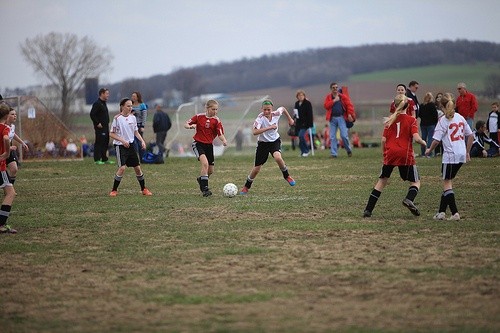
240,186 -> 248,195
348,152 -> 352,158
104,160 -> 115,164
363,210 -> 372,218
433,211 -> 446,220
403,198 -> 420,216
286,176 -> 296,186
329,155 -> 337,159
109,191 -> 118,197
0,224 -> 18,234
448,212 -> 460,221
141,188 -> 152,196
94,160 -> 105,165
202,191 -> 212,197
298,152 -> 310,157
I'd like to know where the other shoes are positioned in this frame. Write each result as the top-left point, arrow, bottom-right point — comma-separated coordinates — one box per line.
412,151 -> 443,159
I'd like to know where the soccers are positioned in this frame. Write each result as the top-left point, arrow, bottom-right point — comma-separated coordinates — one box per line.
223,183 -> 238,197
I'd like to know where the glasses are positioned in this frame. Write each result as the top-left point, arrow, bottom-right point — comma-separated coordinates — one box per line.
457,87 -> 465,90
413,86 -> 418,89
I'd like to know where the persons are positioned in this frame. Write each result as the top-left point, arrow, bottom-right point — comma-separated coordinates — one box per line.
153,104 -> 171,157
292,90 -> 313,156
363,94 -> 427,217
291,123 -> 362,149
405,81 -> 421,119
3,107 -> 28,197
184,99 -> 228,197
454,82 -> 479,147
324,82 -> 353,157
240,98 -> 295,195
389,85 -> 415,117
90,88 -> 116,165
486,102 -> 500,155
418,92 -> 439,157
131,92 -> 147,163
434,92 -> 444,155
21,136 -> 94,158
424,93 -> 474,221
108,97 -> 152,196
470,120 -> 500,158
0,102 -> 17,233
